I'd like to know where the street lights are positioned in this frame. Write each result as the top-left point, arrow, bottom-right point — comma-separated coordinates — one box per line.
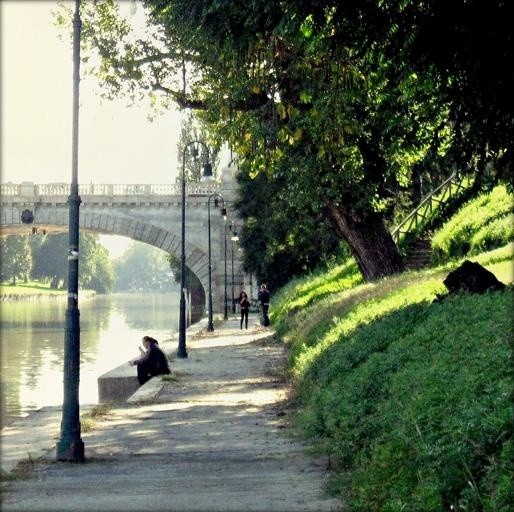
206,193 -> 226,332
177,139 -> 213,360
223,219 -> 239,320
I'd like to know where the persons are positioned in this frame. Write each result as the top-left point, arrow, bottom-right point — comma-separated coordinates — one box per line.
138,336 -> 159,359
258,284 -> 271,327
239,290 -> 250,330
126,337 -> 172,388
